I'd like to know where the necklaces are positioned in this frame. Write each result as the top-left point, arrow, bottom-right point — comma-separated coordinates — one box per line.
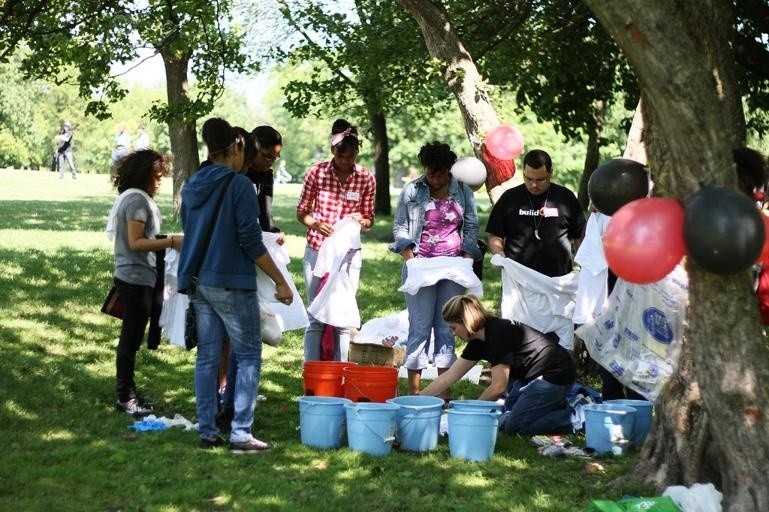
526,188 -> 550,241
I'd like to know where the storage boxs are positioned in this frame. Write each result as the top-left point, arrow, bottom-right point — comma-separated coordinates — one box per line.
347,339 -> 408,369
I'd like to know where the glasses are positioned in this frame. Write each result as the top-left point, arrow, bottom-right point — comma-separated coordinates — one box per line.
522,169 -> 551,184
255,144 -> 281,162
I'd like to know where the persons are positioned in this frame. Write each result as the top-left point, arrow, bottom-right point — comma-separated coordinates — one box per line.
181,118 -> 294,457
111,123 -> 131,167
111,149 -> 186,415
731,145 -> 769,294
57,122 -> 77,179
587,211 -> 650,400
131,122 -> 150,150
484,149 -> 583,363
245,126 -> 283,234
297,118 -> 377,367
198,127 -> 254,431
413,293 -> 593,436
390,141 -> 482,399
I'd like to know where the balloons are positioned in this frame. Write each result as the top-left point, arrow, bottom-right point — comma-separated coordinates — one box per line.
684,183 -> 765,276
450,156 -> 487,191
602,194 -> 684,283
484,126 -> 524,161
587,158 -> 646,214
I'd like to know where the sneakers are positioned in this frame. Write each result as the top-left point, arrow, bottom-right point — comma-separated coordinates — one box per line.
198,432 -> 228,450
230,435 -> 272,450
118,394 -> 152,416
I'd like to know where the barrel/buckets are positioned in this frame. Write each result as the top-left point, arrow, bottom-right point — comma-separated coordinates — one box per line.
343,401 -> 400,456
295,396 -> 352,448
583,403 -> 637,455
603,399 -> 654,451
342,366 -> 398,402
449,399 -> 505,411
445,408 -> 502,462
302,360 -> 358,396
386,394 -> 445,452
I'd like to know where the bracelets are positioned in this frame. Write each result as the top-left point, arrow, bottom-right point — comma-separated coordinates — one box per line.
169,236 -> 174,249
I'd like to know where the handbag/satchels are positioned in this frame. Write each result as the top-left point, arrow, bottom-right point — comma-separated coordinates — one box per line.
542,358 -> 579,385
